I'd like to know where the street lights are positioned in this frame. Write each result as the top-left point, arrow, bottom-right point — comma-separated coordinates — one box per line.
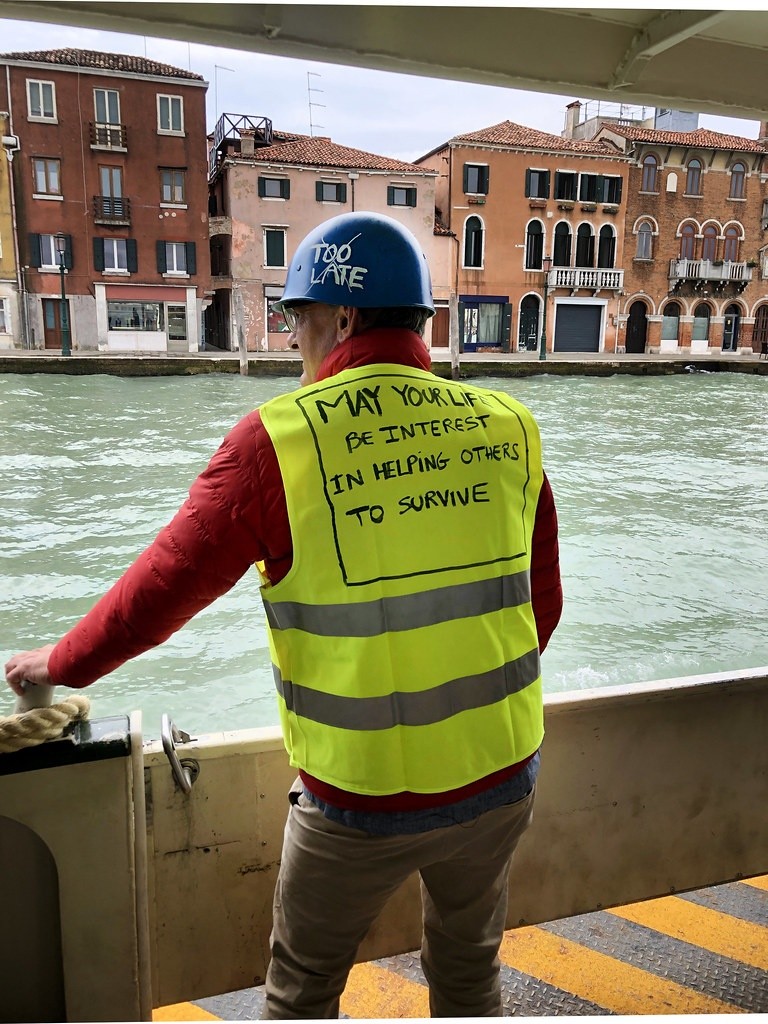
52,231 -> 74,356
538,253 -> 553,361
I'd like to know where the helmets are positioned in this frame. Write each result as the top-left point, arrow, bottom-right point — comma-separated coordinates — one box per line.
271,210 -> 436,317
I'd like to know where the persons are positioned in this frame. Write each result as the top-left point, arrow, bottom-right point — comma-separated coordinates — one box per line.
4,214 -> 564,1020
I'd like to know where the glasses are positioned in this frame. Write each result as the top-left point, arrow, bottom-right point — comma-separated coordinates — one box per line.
281,300 -> 319,333
